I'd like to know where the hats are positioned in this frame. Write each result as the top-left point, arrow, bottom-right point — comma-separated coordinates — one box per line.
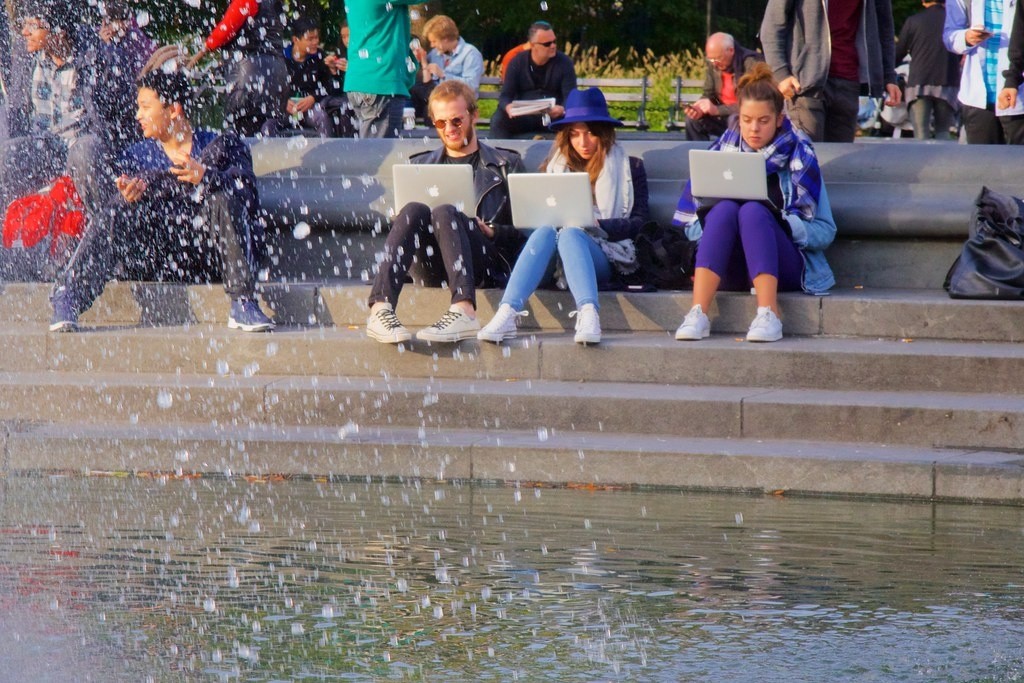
548,87 -> 624,130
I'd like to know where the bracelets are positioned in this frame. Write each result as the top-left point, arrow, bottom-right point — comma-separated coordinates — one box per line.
488,224 -> 495,230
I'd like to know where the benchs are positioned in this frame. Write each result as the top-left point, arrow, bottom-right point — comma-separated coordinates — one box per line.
411,73 -> 651,131
669,78 -> 706,127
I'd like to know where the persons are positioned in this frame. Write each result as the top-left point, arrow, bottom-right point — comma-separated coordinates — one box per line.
501,41 -> 530,84
0,0 -> 483,280
45,68 -> 276,331
488,22 -> 578,140
675,62 -> 837,341
758,0 -> 901,143
476,86 -> 650,343
683,31 -> 766,141
366,80 -> 534,343
857,0 -> 1024,144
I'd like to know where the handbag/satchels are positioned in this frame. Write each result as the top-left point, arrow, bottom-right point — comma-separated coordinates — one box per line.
943,185 -> 1024,300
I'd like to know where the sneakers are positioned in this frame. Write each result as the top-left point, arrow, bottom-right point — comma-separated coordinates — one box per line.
228,299 -> 275,332
746,305 -> 783,341
568,303 -> 601,342
675,304 -> 711,340
366,302 -> 411,343
416,304 -> 481,342
477,303 -> 529,341
49,286 -> 79,332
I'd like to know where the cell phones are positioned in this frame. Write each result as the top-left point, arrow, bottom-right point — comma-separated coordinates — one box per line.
682,103 -> 698,112
970,27 -> 990,34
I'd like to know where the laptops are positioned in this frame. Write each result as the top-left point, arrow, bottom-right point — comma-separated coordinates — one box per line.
392,163 -> 477,218
506,172 -> 609,239
689,149 -> 777,209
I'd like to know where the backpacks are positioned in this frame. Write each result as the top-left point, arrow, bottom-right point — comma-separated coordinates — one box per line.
2,177 -> 90,282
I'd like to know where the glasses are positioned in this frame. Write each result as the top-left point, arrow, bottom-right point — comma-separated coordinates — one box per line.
534,39 -> 557,46
705,54 -> 724,64
432,115 -> 465,128
17,19 -> 46,29
95,18 -> 113,27
